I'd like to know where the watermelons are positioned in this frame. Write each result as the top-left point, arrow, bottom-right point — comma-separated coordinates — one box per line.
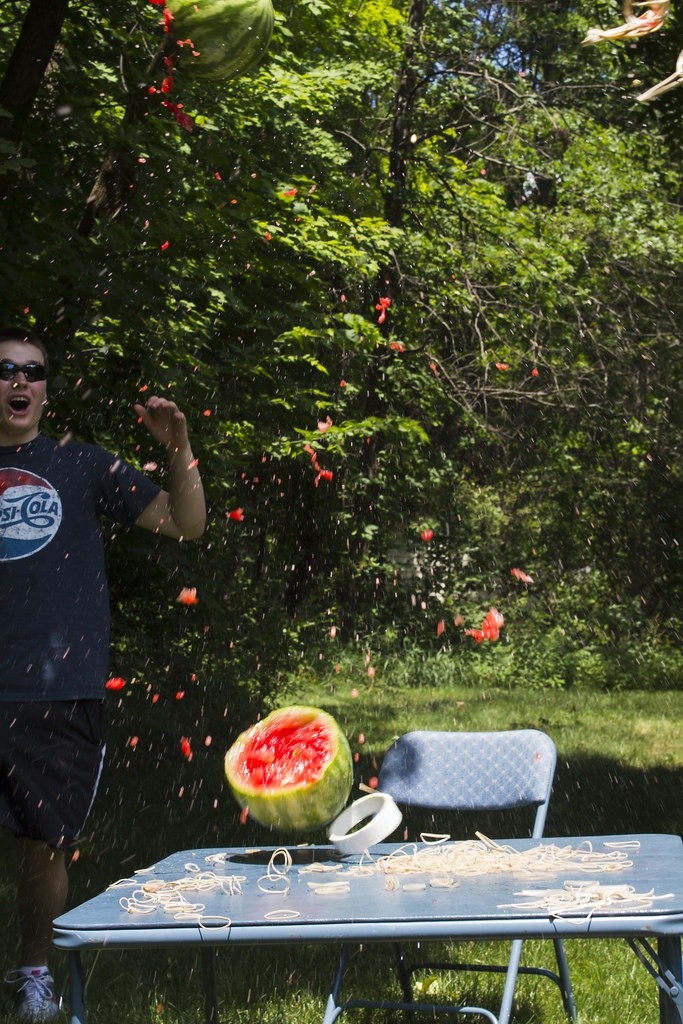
224,705 -> 355,832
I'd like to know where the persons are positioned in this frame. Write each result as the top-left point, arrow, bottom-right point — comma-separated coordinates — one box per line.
0,323 -> 205,1018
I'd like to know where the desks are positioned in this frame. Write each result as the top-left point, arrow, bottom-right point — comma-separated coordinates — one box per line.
49,831 -> 682,1024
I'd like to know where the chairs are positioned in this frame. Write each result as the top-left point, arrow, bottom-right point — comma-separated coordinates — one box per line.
322,728 -> 577,1024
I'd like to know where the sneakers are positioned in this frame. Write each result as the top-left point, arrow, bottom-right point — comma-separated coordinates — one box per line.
4,969 -> 57,1023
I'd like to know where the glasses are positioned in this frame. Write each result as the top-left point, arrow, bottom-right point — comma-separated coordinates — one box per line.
0,358 -> 46,384
